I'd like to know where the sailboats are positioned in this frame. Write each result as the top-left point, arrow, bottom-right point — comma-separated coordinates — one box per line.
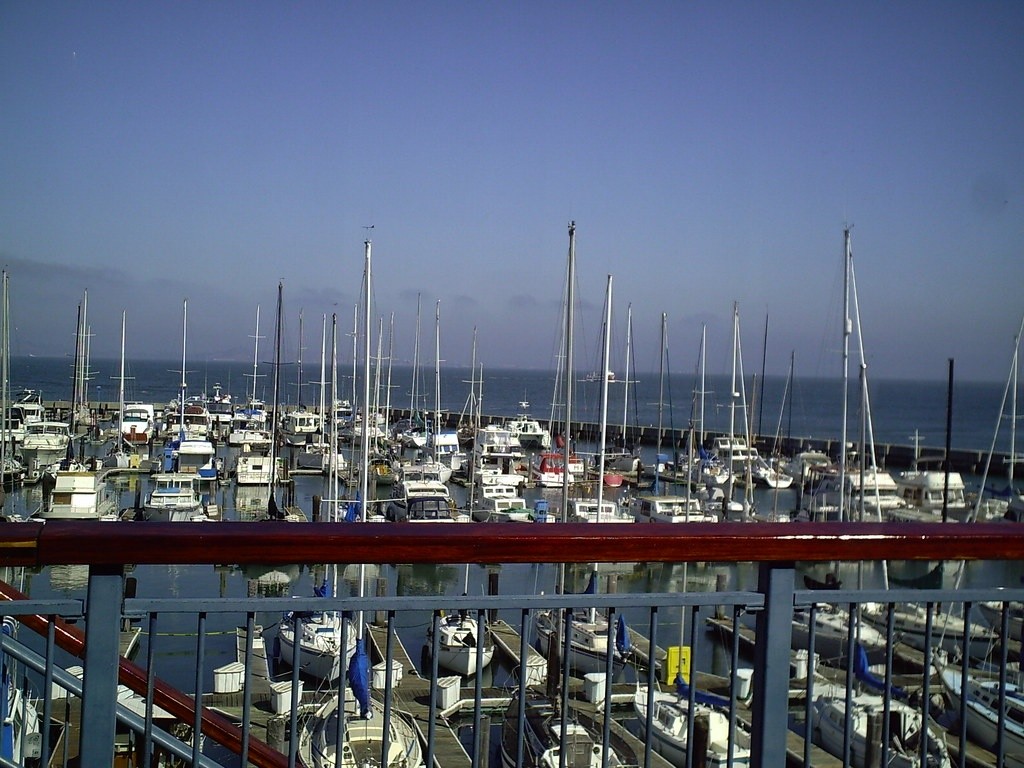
0,219 -> 1024,768
